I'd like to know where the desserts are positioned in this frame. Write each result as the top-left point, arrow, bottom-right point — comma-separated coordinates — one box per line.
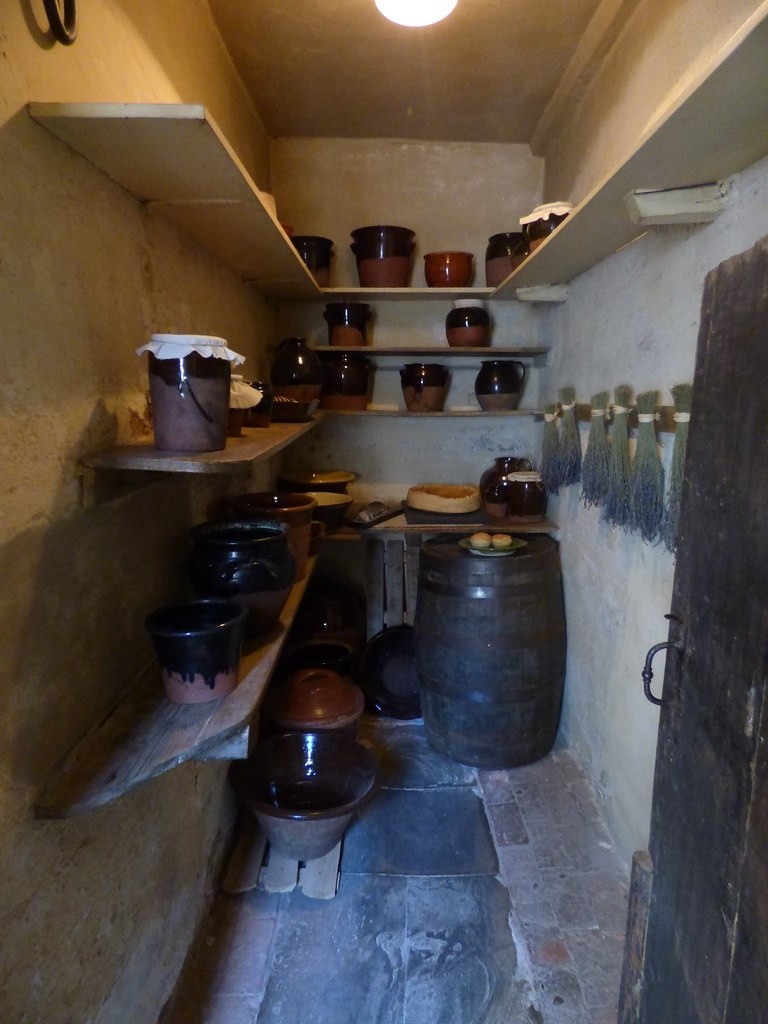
471,532 -> 491,547
492,534 -> 512,547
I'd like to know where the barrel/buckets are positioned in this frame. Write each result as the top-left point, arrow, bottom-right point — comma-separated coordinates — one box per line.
417,533 -> 567,769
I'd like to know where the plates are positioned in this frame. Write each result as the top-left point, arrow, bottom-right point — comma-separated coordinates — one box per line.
460,539 -> 525,557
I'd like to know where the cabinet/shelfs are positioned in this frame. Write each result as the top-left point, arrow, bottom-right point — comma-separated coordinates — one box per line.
28,0 -> 767,820
